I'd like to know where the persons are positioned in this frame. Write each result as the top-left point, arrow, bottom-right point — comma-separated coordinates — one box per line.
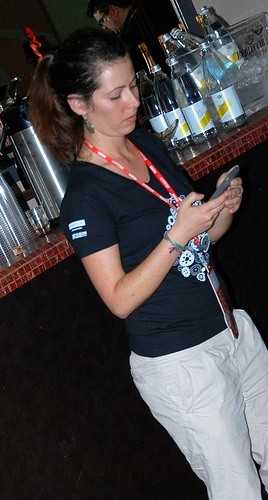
23,28 -> 52,67
87,0 -> 181,74
27,27 -> 268,500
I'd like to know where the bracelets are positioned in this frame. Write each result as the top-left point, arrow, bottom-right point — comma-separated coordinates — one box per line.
164,232 -> 184,256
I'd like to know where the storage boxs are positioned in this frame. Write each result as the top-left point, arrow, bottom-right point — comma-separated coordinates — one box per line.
171,11 -> 268,128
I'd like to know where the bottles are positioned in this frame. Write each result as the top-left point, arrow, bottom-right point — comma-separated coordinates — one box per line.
199,5 -> 245,76
170,28 -> 239,85
162,33 -> 221,101
138,43 -> 156,82
198,42 -> 248,132
136,70 -> 177,152
158,34 -> 201,100
165,55 -> 219,145
195,14 -> 220,53
151,64 -> 194,150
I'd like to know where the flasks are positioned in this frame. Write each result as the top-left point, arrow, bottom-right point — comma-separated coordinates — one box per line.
0,98 -> 71,227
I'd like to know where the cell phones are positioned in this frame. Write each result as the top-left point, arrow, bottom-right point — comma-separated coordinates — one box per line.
210,165 -> 239,200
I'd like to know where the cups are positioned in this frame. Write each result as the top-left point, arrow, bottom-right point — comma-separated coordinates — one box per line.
203,46 -> 267,123
0,172 -> 36,267
25,204 -> 52,237
